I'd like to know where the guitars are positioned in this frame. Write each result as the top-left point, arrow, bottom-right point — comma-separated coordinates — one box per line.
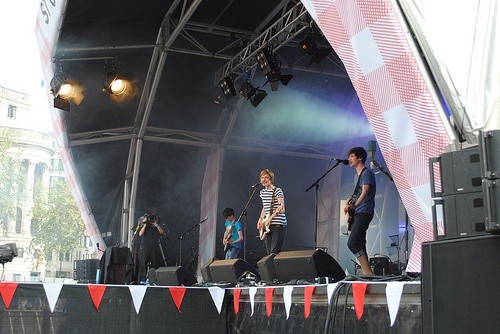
222,219 -> 236,245
257,204 -> 284,240
347,195 -> 355,231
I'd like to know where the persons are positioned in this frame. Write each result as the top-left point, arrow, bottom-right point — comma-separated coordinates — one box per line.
255,169 -> 288,256
137,213 -> 164,285
344,146 -> 376,278
222,207 -> 244,260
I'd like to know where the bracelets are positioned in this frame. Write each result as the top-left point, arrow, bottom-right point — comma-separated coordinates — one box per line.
155,223 -> 158,227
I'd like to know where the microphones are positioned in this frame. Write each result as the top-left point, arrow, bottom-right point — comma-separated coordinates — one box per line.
96,243 -> 99,252
200,216 -> 208,223
368,140 -> 376,170
333,158 -> 349,165
252,181 -> 264,188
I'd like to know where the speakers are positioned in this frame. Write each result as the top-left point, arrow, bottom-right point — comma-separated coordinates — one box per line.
274,249 -> 346,284
156,266 -> 193,286
421,235 -> 500,334
209,258 -> 261,285
201,262 -> 212,283
258,254 -> 276,282
